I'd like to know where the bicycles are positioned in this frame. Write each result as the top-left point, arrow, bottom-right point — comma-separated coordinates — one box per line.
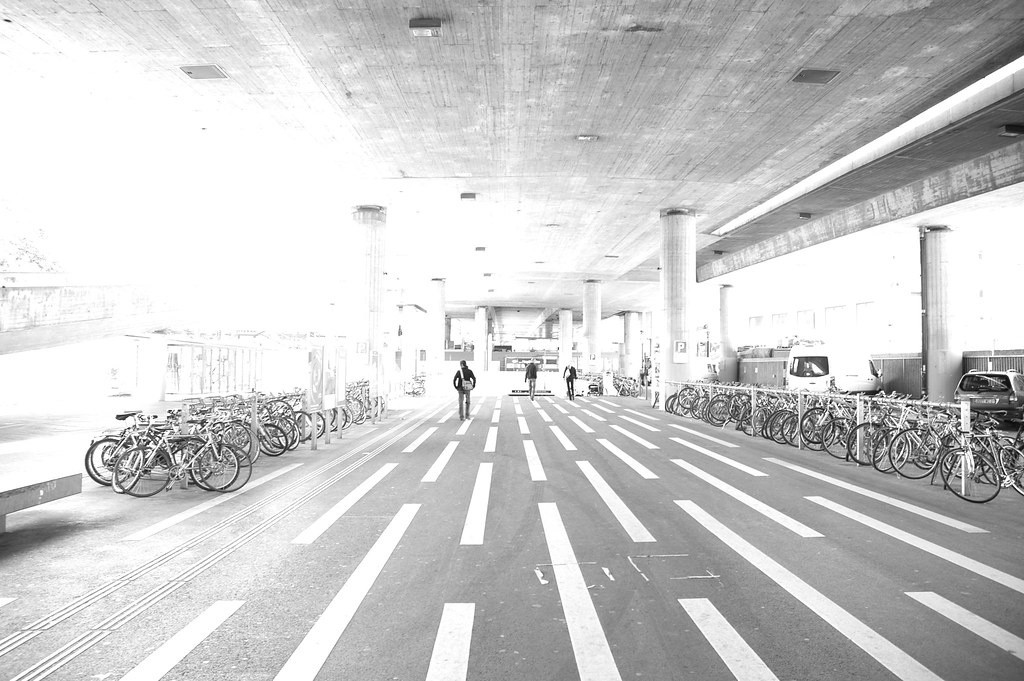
85,376 -> 384,498
585,372 -> 644,398
403,371 -> 428,397
665,378 -> 1024,504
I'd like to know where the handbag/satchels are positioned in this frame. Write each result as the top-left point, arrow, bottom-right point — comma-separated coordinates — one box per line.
462,379 -> 473,390
565,369 -> 571,380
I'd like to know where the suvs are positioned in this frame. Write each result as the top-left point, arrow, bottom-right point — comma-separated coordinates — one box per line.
953,369 -> 1024,429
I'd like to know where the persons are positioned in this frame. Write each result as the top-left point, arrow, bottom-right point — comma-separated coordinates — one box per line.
525,358 -> 537,402
453,360 -> 476,421
563,362 -> 577,401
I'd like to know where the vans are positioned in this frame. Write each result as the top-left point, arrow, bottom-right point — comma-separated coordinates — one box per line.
786,343 -> 884,394
